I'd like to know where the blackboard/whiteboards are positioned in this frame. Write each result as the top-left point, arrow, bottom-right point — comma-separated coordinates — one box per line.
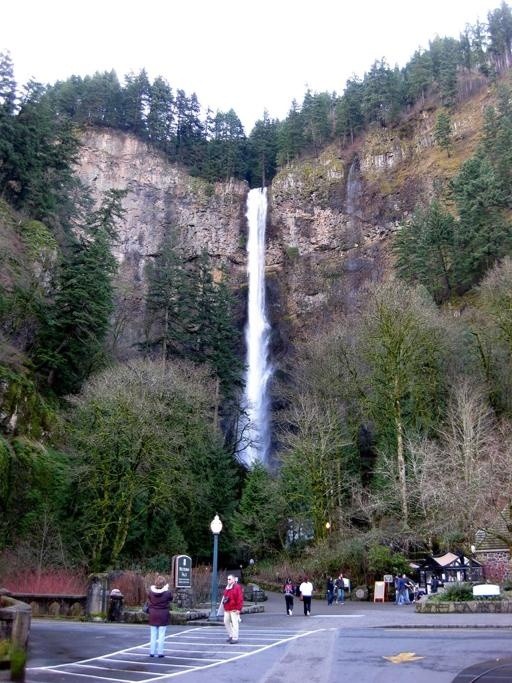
375,582 -> 385,599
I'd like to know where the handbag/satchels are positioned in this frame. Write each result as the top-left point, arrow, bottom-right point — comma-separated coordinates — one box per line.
142,600 -> 149,613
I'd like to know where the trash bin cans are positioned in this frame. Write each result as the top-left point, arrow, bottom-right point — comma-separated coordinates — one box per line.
111,589 -> 124,623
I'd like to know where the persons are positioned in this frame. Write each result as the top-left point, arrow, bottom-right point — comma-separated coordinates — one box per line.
299,575 -> 314,616
282,576 -> 297,616
430,574 -> 437,593
325,574 -> 335,607
220,572 -> 244,645
335,572 -> 345,605
399,573 -> 418,604
145,575 -> 174,658
393,575 -> 410,604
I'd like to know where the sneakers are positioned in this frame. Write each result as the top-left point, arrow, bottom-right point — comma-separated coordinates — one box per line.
286,609 -> 310,616
230,640 -> 238,644
226,637 -> 232,642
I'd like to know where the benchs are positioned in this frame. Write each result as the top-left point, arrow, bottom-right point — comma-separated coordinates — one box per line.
369,592 -> 394,602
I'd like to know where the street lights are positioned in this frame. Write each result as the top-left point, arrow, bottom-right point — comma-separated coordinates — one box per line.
208,512 -> 223,622
250,559 -> 254,602
